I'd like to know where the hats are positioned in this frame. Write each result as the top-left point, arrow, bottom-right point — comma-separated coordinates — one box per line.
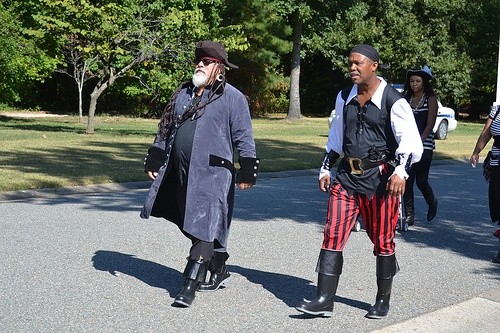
195,40 -> 239,69
406,64 -> 433,82
351,44 -> 380,62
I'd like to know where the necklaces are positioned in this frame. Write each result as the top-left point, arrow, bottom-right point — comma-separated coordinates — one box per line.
409,93 -> 426,114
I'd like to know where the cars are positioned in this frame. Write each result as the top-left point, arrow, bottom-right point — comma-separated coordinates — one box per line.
329,85 -> 457,140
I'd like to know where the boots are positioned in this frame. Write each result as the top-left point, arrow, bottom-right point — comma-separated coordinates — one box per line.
174,258 -> 208,308
295,249 -> 343,317
422,188 -> 437,221
404,200 -> 415,225
198,251 -> 231,291
366,252 -> 400,319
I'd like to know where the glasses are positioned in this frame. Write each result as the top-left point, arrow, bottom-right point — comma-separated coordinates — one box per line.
194,59 -> 220,66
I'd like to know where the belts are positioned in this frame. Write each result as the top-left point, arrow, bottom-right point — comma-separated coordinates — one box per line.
342,149 -> 396,176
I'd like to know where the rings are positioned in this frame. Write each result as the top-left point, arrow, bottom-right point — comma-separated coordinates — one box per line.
398,188 -> 401,191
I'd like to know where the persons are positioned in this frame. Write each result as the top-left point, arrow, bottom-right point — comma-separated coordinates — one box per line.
139,41 -> 260,308
470,104 -> 500,263
401,63 -> 438,226
296,43 -> 424,319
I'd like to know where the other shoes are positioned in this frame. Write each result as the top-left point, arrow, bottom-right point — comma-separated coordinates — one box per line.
493,249 -> 500,263
